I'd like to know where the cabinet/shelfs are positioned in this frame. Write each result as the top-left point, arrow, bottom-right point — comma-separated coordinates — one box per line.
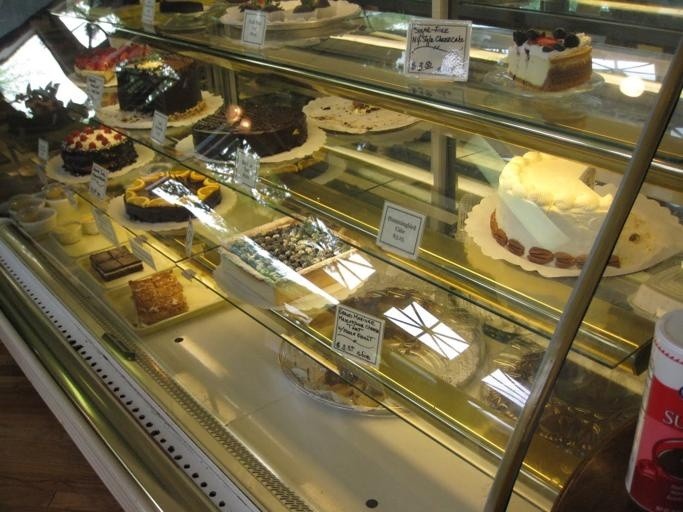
0,0 -> 683,512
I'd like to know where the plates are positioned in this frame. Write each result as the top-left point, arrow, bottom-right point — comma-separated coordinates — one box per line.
100,266 -> 228,334
482,68 -> 605,98
219,211 -> 363,287
76,239 -> 169,290
275,286 -> 486,413
217,4 -> 361,34
217,22 -> 362,50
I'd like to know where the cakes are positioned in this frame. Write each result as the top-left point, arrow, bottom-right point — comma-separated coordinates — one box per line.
292,287 -> 478,407
482,350 -> 640,456
60,125 -> 137,172
235,0 -> 285,22
8,183 -> 98,245
127,272 -> 186,324
258,148 -> 331,184
191,92 -> 307,159
73,44 -> 151,84
87,245 -> 144,281
122,169 -> 220,223
290,1 -> 336,18
116,54 -> 202,120
489,151 -> 659,271
505,27 -> 591,92
157,0 -> 203,16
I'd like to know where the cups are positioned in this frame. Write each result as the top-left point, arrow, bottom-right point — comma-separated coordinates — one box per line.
623,308 -> 683,511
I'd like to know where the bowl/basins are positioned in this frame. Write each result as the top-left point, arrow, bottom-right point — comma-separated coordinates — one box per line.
32,192 -> 68,213
7,197 -> 44,221
15,208 -> 56,236
52,220 -> 81,246
76,212 -> 99,236
